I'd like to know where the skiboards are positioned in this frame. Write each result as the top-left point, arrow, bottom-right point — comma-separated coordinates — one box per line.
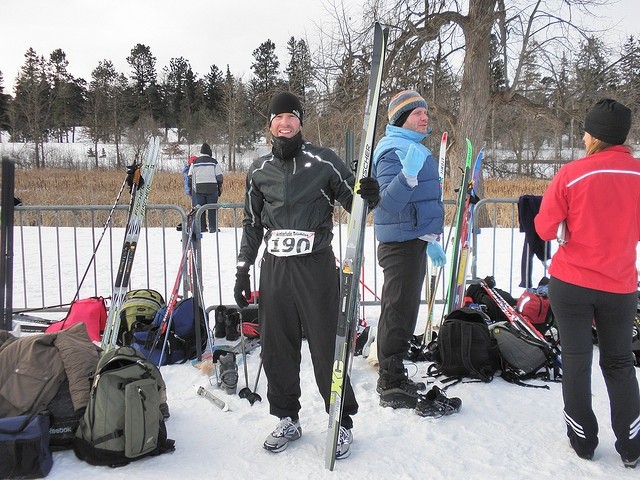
480,276 -> 564,369
325,23 -> 389,471
421,133 -> 454,346
101,136 -> 160,357
448,139 -> 486,314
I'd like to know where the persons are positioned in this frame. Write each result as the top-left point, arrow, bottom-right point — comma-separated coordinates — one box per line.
371,89 -> 447,412
188,143 -> 223,233
234,92 -> 381,460
181,156 -> 192,207
533,98 -> 640,467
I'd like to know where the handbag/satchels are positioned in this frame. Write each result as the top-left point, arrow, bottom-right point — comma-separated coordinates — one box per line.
0,413 -> 54,480
466,275 -> 517,322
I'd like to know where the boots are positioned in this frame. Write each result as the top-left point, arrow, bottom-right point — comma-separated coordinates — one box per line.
215,306 -> 227,337
226,308 -> 241,341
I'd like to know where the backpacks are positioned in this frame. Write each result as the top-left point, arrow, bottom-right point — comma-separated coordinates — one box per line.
73,345 -> 177,469
516,290 -> 554,336
47,379 -> 89,453
488,321 -> 563,389
45,296 -> 107,344
126,298 -> 208,367
427,308 -> 500,386
102,288 -> 166,346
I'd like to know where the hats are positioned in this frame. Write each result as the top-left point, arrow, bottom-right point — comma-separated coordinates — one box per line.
387,90 -> 427,127
200,143 -> 212,157
188,155 -> 198,164
268,92 -> 303,128
582,97 -> 632,146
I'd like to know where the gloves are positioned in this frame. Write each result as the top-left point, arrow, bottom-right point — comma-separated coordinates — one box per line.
426,242 -> 448,267
357,177 -> 380,212
234,266 -> 252,309
218,186 -> 222,196
394,143 -> 426,177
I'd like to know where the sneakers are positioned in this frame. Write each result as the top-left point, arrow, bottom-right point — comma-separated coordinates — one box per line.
622,456 -> 640,471
380,383 -> 421,408
262,416 -> 302,453
336,425 -> 353,460
414,385 -> 462,418
377,376 -> 426,393
